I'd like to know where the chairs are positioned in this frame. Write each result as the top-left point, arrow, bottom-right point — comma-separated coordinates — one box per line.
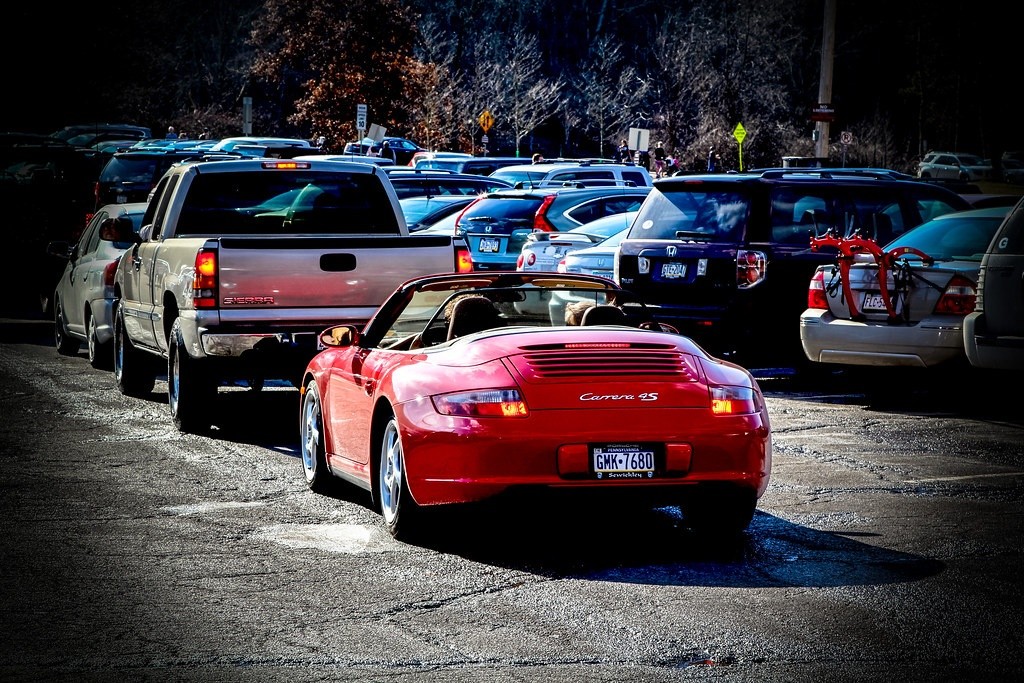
446,296 -> 500,342
581,305 -> 627,325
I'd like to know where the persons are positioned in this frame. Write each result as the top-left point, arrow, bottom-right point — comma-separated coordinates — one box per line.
408,295 -> 483,350
165,126 -> 544,165
565,300 -> 599,326
617,139 -> 720,178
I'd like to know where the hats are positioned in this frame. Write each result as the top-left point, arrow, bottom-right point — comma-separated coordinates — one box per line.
383,141 -> 389,146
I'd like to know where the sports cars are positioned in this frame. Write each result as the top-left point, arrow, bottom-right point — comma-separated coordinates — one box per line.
297,271 -> 775,553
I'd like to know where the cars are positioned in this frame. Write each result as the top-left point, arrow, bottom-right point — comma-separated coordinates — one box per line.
915,153 -> 995,183
1,120 -> 1024,403
50,202 -> 156,367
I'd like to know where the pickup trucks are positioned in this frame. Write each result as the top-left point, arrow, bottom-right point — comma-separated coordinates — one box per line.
108,157 -> 478,430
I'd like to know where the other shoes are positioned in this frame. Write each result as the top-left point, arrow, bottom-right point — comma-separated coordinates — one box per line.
656,175 -> 660,178
